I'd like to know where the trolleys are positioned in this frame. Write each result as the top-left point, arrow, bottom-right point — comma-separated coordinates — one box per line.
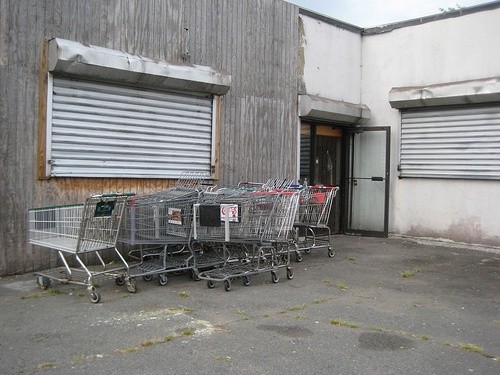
25,174 -> 340,306
